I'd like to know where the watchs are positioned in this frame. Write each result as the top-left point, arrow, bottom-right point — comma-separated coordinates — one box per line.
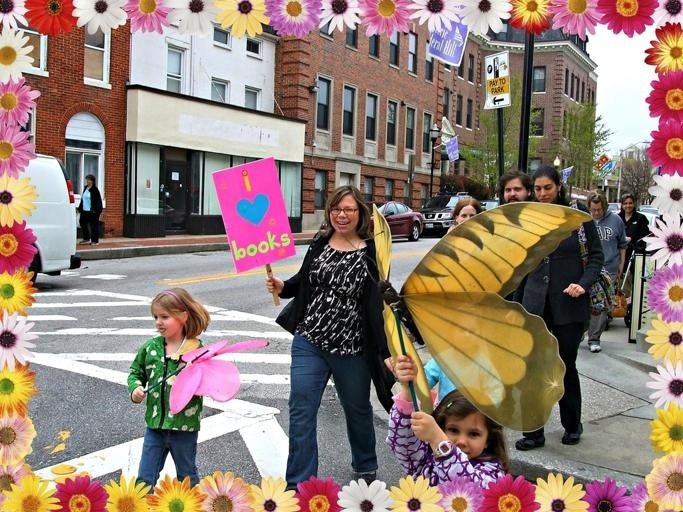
430,440 -> 455,457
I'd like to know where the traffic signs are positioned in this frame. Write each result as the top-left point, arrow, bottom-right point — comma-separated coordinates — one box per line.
482,49 -> 510,111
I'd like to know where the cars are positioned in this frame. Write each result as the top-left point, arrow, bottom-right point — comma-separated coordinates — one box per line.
478,199 -> 498,212
607,201 -> 661,228
133,197 -> 185,225
319,201 -> 424,242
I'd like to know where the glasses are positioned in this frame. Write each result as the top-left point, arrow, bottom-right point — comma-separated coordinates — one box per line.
329,205 -> 358,217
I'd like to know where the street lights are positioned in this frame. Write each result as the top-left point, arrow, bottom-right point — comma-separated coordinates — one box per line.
615,141 -> 651,202
428,123 -> 439,197
553,156 -> 560,171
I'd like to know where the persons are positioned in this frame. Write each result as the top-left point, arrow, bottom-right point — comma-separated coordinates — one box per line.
74,174 -> 104,246
266,184 -> 381,499
512,164 -> 604,453
615,192 -> 649,329
126,287 -> 212,497
451,196 -> 482,227
494,167 -> 541,421
574,189 -> 627,354
383,353 -> 508,493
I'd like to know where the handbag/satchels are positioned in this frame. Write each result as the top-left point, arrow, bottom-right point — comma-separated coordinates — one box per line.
275,298 -> 298,335
611,288 -> 627,318
588,268 -> 616,316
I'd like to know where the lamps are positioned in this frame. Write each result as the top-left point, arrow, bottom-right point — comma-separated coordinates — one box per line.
307,78 -> 320,94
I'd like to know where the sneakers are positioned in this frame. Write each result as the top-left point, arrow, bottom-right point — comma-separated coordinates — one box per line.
79,240 -> 99,246
589,343 -> 602,353
516,435 -> 545,451
562,423 -> 583,446
358,471 -> 376,484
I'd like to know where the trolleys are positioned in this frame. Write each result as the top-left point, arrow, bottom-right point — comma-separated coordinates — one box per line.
604,244 -> 637,330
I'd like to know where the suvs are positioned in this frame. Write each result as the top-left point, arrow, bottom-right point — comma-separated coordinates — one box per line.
419,191 -> 473,238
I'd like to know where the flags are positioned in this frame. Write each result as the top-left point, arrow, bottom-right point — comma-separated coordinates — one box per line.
426,16 -> 470,68
444,135 -> 460,163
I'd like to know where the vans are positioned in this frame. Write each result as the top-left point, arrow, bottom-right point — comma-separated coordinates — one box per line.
15,153 -> 83,286
